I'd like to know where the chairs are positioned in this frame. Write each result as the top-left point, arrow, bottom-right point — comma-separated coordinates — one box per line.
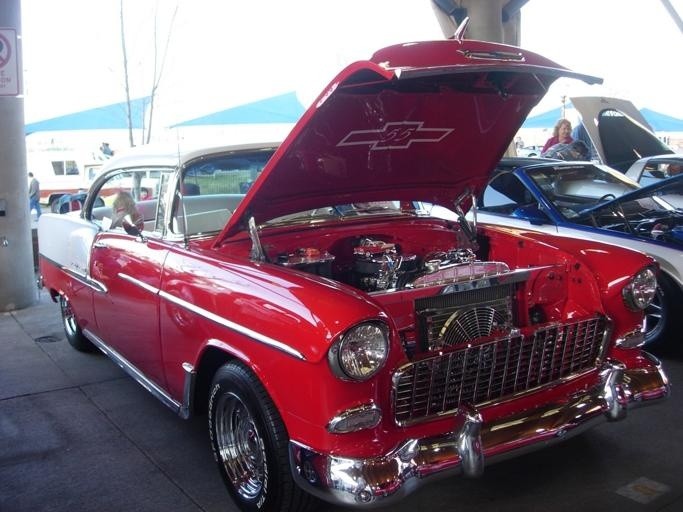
173,208 -> 233,235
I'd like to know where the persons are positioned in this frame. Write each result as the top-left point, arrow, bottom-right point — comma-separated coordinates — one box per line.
27,172 -> 41,222
571,121 -> 600,160
515,136 -> 524,156
540,140 -> 589,162
541,119 -> 574,154
